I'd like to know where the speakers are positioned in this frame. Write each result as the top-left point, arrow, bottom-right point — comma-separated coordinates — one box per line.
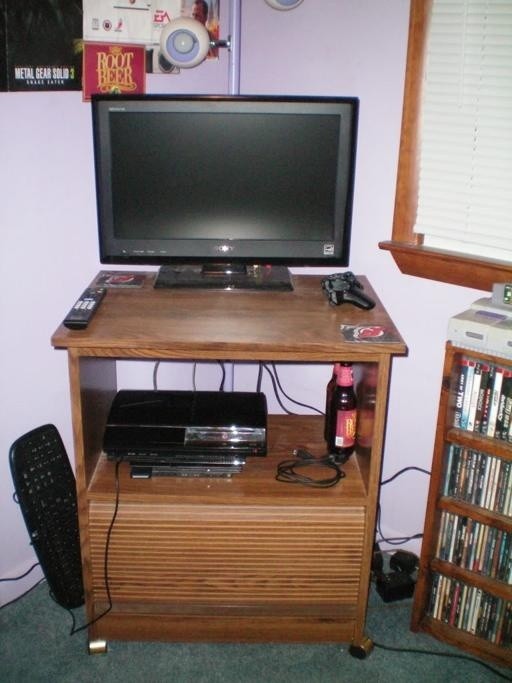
160,16 -> 210,69
265,0 -> 304,11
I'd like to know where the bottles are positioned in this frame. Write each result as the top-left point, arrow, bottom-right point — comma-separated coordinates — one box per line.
322,360 -> 359,463
355,362 -> 378,458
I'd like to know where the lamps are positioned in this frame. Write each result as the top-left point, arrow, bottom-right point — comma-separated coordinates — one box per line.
159,0 -> 302,94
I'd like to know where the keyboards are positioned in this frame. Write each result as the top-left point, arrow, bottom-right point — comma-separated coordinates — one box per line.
8,424 -> 86,606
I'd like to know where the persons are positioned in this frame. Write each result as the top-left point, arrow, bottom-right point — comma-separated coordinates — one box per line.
190,0 -> 218,55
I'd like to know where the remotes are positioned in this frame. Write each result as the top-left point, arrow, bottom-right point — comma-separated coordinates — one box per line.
64,287 -> 107,328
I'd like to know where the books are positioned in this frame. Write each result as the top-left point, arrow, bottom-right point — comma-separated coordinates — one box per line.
423,352 -> 512,652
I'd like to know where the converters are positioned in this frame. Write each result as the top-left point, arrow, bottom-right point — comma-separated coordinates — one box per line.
376,574 -> 414,601
389,548 -> 419,574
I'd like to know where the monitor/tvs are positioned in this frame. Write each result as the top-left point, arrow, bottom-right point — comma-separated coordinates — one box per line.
91,94 -> 359,290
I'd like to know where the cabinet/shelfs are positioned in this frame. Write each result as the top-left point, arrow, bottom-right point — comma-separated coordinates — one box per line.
49,269 -> 408,662
408,341 -> 511,672
